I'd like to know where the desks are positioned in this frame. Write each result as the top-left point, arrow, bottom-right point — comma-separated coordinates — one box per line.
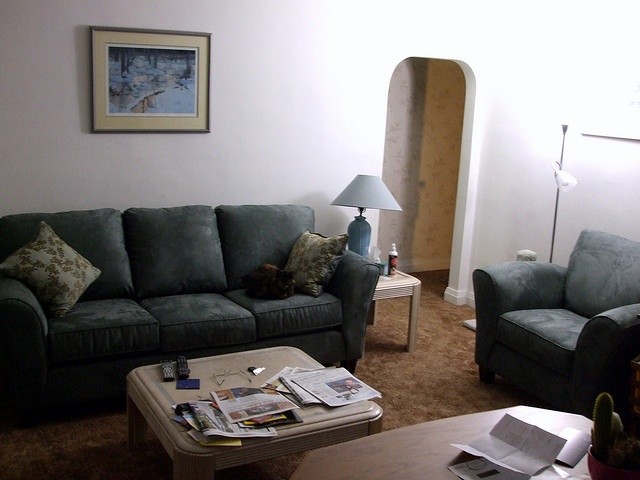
356,269 -> 421,358
289,405 -> 594,480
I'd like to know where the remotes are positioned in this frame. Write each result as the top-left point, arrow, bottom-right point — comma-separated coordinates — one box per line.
176,356 -> 190,379
160,359 -> 175,382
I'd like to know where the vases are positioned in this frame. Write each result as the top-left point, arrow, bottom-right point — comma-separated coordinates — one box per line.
586,392 -> 639,479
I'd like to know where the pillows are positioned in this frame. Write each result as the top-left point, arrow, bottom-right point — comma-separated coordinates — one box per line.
284,229 -> 350,298
1,221 -> 101,320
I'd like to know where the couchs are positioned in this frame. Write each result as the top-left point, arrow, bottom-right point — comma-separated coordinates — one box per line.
472,226 -> 640,422
1,204 -> 380,430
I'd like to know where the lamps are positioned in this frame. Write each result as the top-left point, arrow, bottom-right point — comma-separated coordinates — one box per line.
329,175 -> 403,258
547,108 -> 578,263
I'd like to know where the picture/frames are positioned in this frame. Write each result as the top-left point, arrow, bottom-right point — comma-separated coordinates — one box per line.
88,25 -> 212,134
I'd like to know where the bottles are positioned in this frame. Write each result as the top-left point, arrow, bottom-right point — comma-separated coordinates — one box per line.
386,243 -> 398,276
